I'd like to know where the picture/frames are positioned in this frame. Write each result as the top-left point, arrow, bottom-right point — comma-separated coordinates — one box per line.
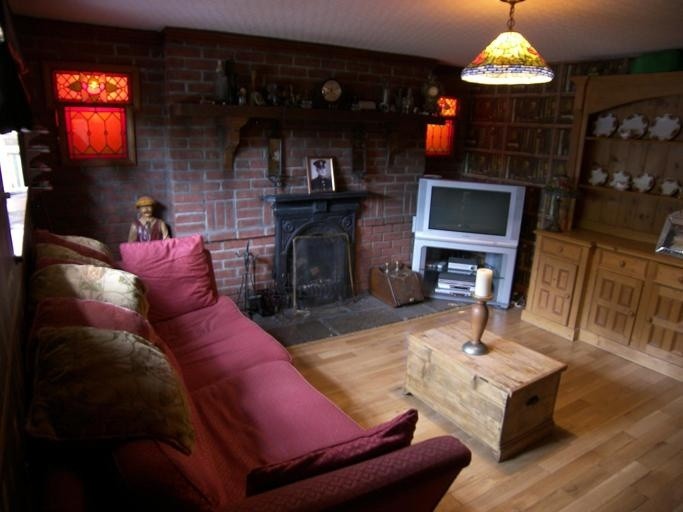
306,153 -> 336,193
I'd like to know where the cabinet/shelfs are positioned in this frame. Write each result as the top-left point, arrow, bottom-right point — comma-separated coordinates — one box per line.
457,59 -> 683,385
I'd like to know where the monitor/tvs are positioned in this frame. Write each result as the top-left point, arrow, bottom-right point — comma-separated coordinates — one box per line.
415,178 -> 526,249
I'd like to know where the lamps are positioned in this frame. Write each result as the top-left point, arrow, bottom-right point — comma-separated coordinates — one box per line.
459,1 -> 554,86
461,267 -> 494,354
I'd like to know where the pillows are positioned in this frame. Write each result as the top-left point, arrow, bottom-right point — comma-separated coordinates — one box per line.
25,228 -> 414,495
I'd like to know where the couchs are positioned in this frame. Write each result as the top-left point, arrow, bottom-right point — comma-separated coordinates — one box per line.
25,229 -> 471,512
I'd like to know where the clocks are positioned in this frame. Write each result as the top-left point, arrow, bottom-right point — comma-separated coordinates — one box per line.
321,80 -> 342,102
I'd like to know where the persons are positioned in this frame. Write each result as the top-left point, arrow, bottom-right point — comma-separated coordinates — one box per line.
127,195 -> 171,242
311,160 -> 332,190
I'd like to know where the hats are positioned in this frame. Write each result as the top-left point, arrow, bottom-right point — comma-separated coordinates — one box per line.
313,160 -> 326,168
